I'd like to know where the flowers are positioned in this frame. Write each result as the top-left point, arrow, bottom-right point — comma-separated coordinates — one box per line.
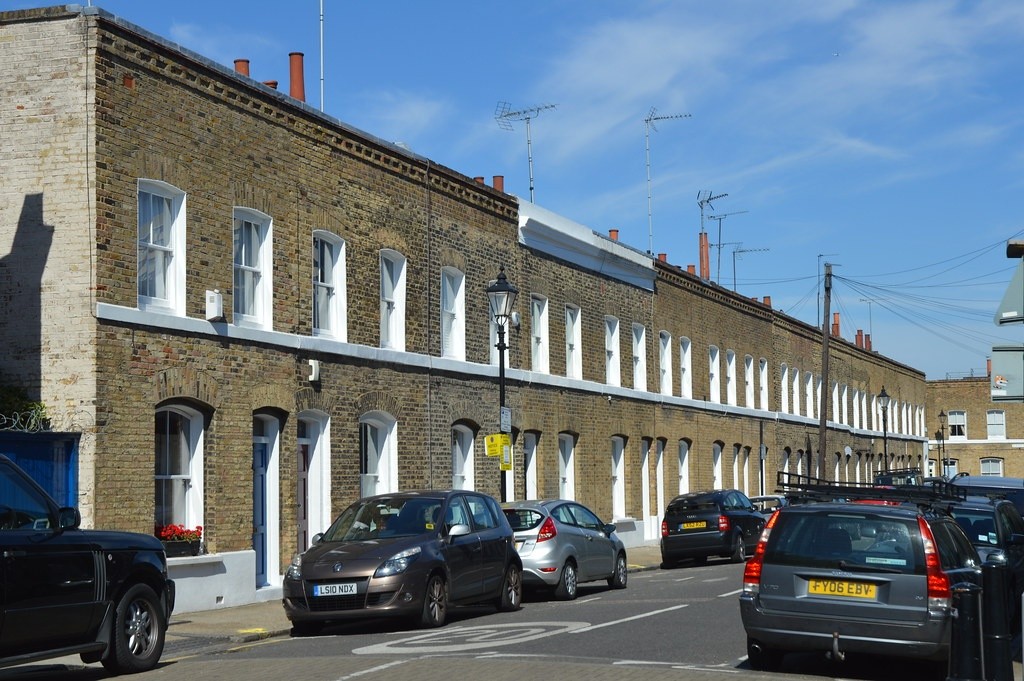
156,524 -> 202,541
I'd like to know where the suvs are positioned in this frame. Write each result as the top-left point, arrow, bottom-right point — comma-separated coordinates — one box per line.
948,472 -> 1024,524
737,500 -> 988,675
930,492 -> 1024,644
1,451 -> 176,674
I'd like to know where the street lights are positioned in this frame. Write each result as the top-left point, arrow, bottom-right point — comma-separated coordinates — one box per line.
875,385 -> 892,473
935,429 -> 944,477
937,408 -> 949,476
485,262 -> 519,504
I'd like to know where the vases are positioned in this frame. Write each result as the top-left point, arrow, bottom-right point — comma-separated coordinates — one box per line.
163,541 -> 201,557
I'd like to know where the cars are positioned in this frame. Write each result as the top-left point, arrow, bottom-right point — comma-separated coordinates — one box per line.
660,489 -> 768,564
282,485 -> 529,632
472,498 -> 629,598
